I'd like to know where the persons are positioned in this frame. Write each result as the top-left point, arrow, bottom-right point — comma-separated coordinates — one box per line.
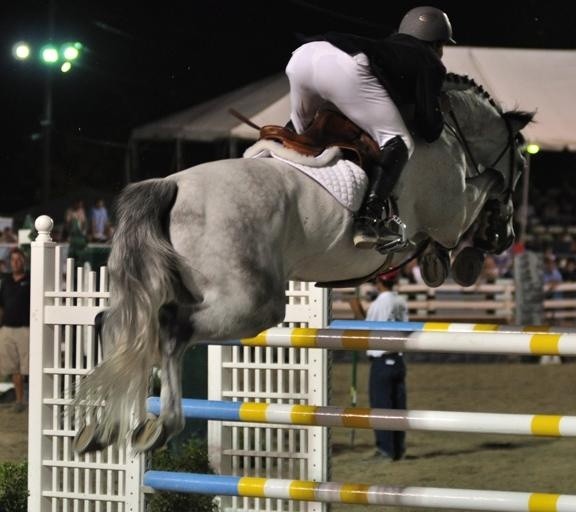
0,200 -> 116,273
476,248 -> 576,324
357,279 -> 379,313
362,268 -> 409,463
0,248 -> 31,413
283,6 -> 457,252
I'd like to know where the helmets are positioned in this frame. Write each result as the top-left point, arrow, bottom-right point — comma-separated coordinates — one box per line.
398,6 -> 456,46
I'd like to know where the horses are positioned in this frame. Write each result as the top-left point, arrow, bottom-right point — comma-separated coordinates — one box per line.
61,71 -> 538,457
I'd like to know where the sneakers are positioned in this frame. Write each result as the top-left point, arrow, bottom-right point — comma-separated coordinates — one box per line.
363,448 -> 393,462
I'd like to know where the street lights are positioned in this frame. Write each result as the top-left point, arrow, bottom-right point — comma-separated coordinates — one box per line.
9,1 -> 89,217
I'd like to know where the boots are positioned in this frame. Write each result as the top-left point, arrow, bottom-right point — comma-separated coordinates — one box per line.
352,134 -> 416,253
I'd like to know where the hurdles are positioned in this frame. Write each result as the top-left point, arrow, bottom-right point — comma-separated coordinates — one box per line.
201,278 -> 576,512
27,215 -> 576,512
